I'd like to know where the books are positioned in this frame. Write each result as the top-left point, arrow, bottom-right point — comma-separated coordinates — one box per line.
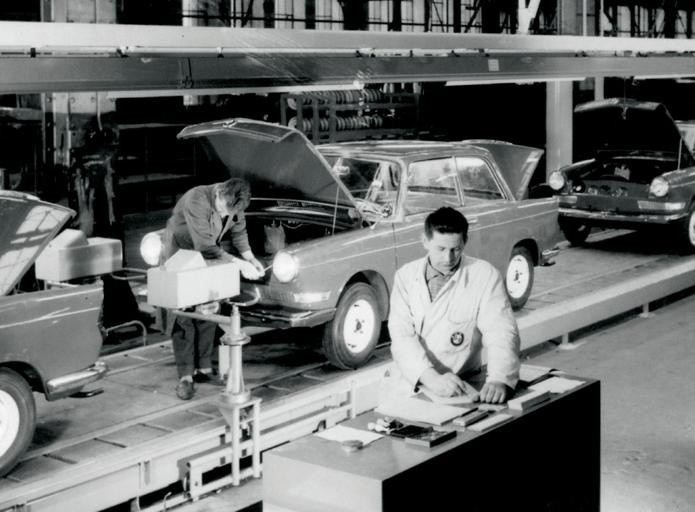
519,364 -> 561,385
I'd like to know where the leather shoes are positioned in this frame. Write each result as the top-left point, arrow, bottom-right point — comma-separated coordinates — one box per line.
176,378 -> 194,400
194,369 -> 227,385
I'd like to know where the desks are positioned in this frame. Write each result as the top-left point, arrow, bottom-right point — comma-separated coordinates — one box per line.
262,363 -> 601,512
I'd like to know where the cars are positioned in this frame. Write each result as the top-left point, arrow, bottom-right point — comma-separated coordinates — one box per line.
140,116 -> 561,372
0,190 -> 109,484
547,97 -> 694,251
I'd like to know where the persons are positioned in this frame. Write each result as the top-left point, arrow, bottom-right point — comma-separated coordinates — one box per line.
387,207 -> 520,404
161,178 -> 266,399
